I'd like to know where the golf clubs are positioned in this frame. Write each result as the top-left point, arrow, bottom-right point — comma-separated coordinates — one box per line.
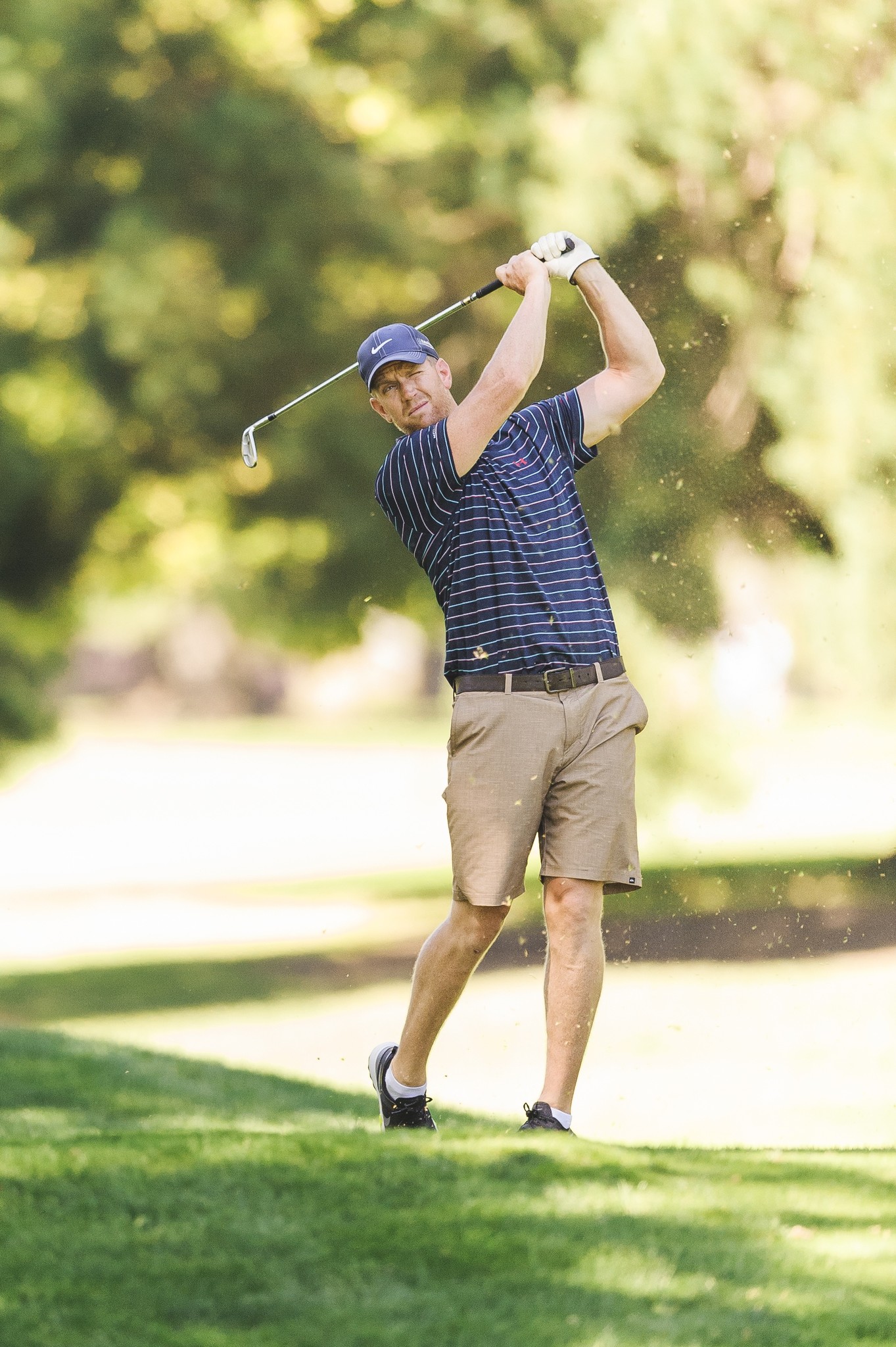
241,236 -> 576,469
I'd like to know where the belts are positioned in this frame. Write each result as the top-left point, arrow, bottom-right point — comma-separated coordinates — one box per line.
455,656 -> 626,694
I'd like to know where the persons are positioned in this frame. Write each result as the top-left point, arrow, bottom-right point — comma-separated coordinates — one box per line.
358,233 -> 667,1133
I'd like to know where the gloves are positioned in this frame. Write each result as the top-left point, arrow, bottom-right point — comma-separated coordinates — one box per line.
530,230 -> 600,286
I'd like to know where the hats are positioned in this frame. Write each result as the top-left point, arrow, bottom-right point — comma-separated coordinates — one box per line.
357,323 -> 438,393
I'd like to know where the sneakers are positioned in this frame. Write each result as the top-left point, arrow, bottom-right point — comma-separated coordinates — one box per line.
368,1042 -> 438,1130
518,1101 -> 574,1135
